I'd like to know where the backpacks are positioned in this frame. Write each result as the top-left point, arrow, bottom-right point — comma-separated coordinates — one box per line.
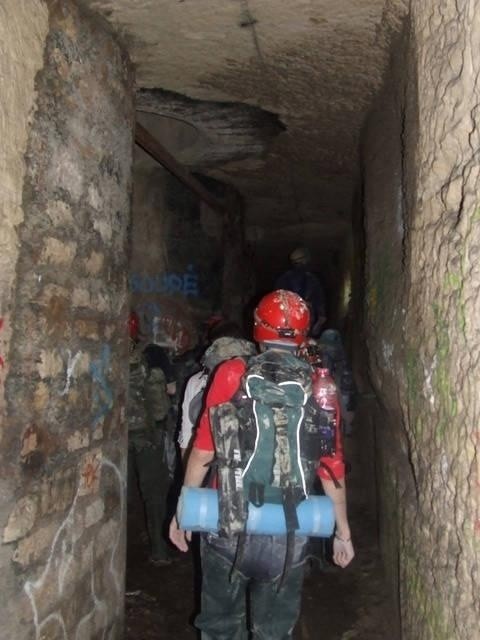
236,348 -> 318,505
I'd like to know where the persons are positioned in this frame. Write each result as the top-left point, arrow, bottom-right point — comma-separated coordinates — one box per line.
166,289 -> 356,640
129,300 -> 373,572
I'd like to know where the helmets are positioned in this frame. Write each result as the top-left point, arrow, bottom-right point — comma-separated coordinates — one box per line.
202,337 -> 256,372
253,289 -> 311,344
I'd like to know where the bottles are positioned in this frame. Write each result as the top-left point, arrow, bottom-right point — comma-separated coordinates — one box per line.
313,367 -> 338,450
